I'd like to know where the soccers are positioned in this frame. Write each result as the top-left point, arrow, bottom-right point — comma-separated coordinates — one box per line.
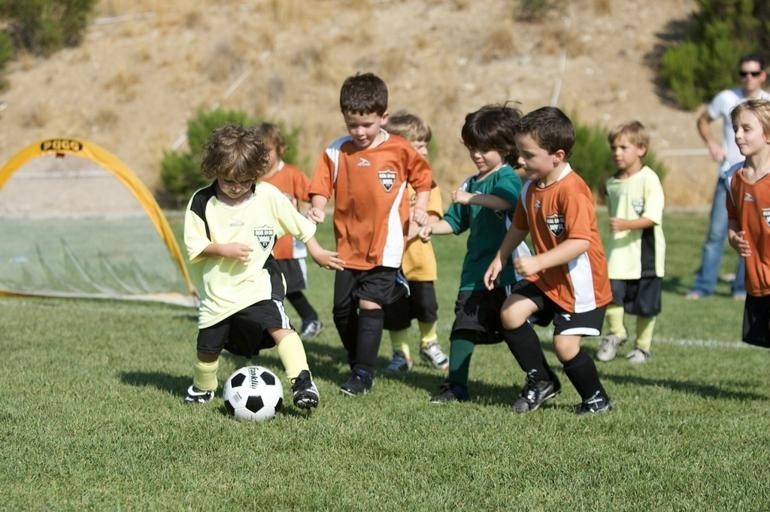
224,366 -> 284,423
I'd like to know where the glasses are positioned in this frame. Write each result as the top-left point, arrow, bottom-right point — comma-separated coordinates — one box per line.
740,70 -> 762,77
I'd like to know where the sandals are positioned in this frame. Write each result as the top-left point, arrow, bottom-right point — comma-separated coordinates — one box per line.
686,292 -> 701,300
733,294 -> 746,299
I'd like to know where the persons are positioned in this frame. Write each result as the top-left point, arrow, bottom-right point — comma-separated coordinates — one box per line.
595,121 -> 665,363
305,73 -> 432,397
484,107 -> 614,417
184,125 -> 345,414
417,106 -> 534,404
687,55 -> 770,298
383,115 -> 449,376
725,101 -> 770,348
250,125 -> 324,337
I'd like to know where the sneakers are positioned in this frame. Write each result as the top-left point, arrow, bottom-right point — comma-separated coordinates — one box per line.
297,320 -> 323,336
514,369 -> 561,412
291,371 -> 320,411
596,333 -> 628,362
574,391 -> 612,415
185,385 -> 216,403
624,349 -> 650,363
430,386 -> 469,404
387,356 -> 412,375
420,342 -> 450,370
339,370 -> 371,397
